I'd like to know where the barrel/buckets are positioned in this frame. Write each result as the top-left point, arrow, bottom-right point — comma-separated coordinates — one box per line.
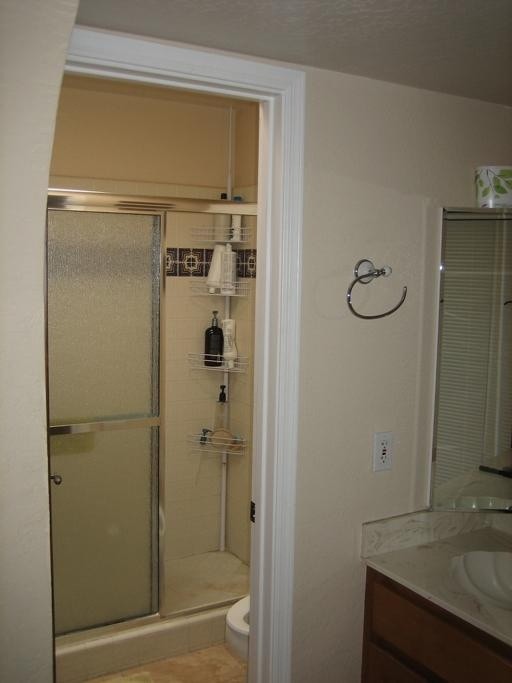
474,165 -> 511,207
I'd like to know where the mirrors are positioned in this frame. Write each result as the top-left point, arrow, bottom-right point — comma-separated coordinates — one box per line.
431,218 -> 512,514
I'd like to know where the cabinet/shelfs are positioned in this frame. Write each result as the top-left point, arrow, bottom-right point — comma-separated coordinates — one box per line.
184,220 -> 252,459
359,569 -> 512,682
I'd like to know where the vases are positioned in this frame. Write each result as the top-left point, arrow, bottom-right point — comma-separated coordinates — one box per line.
474,163 -> 512,210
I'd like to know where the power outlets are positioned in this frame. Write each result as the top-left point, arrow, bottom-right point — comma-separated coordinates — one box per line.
372,430 -> 395,472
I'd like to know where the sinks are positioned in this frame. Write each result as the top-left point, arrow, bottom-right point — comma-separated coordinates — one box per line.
454,476 -> 511,511
458,549 -> 511,612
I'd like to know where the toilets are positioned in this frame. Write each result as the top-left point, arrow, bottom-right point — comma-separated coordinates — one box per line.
224,595 -> 251,671
